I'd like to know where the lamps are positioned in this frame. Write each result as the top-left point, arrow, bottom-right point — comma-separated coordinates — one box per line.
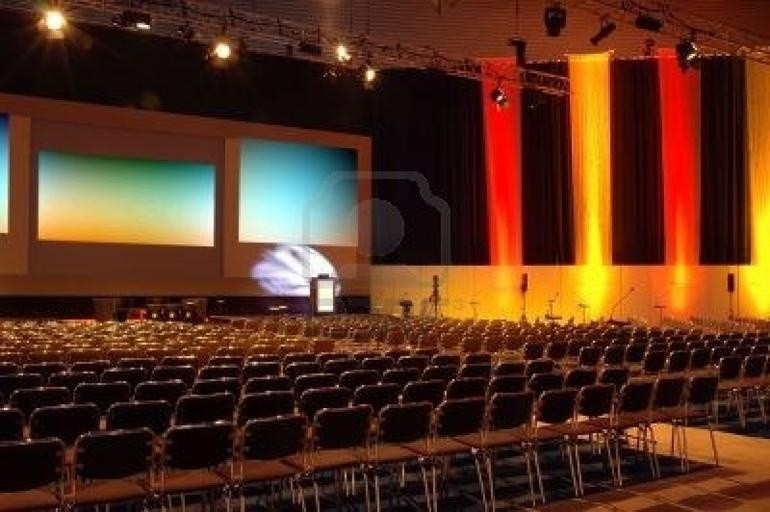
489,0 -> 716,106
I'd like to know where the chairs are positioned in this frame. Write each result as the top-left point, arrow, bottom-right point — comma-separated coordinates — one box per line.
0,308 -> 770,512
